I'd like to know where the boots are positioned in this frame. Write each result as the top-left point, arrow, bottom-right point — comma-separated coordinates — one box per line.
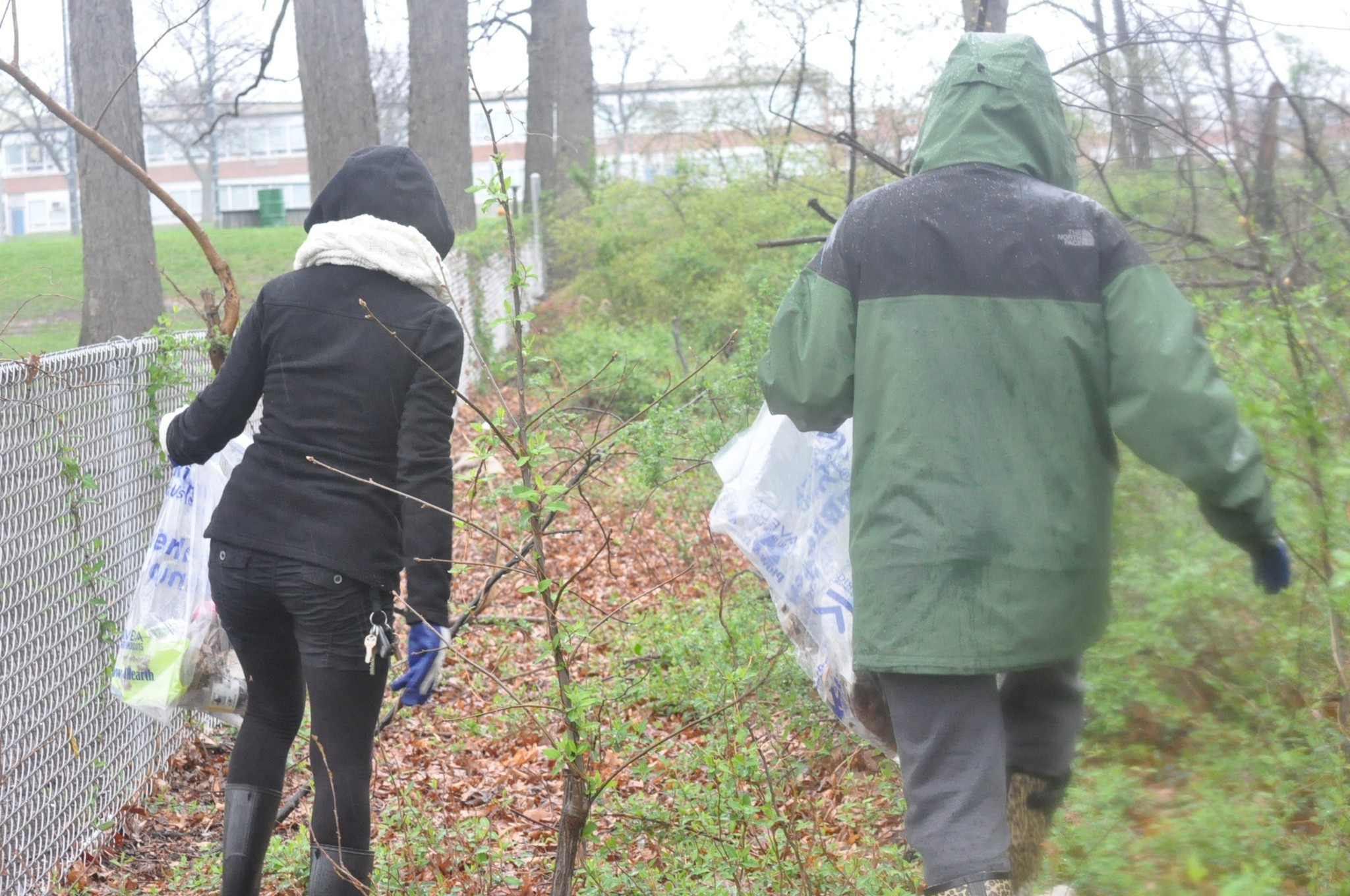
218,783 -> 282,896
923,871 -> 1015,896
308,843 -> 375,896
1005,769 -> 1064,896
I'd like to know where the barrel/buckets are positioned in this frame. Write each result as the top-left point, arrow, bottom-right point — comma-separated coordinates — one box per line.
258,188 -> 287,227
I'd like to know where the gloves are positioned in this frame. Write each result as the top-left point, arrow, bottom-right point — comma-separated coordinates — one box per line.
159,406 -> 185,459
389,621 -> 451,706
1250,533 -> 1292,595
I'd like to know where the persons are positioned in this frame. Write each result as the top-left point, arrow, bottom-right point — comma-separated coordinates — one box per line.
160,147 -> 465,895
760,32 -> 1290,896
